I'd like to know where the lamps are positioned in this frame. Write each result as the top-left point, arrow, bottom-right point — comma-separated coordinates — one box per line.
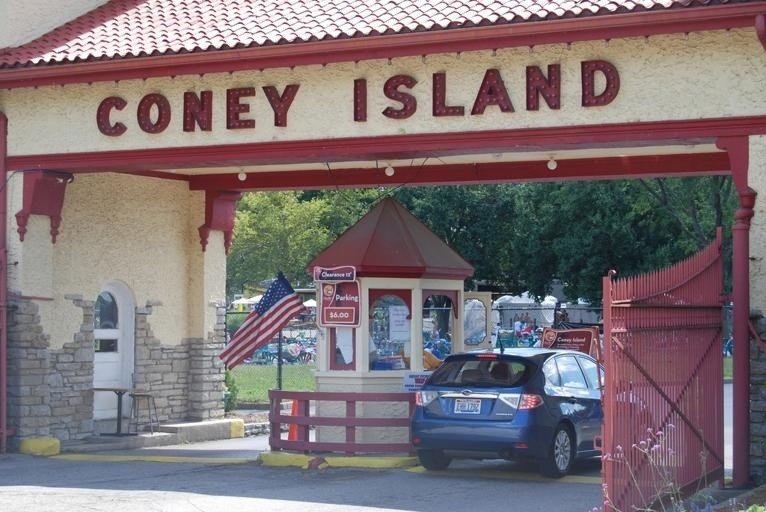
385,160 -> 395,176
547,154 -> 557,170
238,168 -> 247,181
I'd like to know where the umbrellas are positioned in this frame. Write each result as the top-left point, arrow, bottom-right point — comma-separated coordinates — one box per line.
232,297 -> 254,311
303,299 -> 317,307
248,295 -> 264,303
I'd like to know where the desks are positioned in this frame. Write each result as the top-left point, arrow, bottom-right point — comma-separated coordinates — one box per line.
93,387 -> 145,436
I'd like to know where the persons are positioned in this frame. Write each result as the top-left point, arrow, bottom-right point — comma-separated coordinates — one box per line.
430,326 -> 452,354
532,328 -> 543,347
514,312 -> 530,324
555,303 -> 569,324
599,316 -> 603,324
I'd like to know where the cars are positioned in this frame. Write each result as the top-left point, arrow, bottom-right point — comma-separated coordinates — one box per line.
410,337 -> 652,478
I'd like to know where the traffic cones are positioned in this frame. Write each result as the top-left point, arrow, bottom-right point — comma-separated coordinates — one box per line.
288,400 -> 298,441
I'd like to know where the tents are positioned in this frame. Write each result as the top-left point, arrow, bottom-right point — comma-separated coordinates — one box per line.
503,290 -> 557,329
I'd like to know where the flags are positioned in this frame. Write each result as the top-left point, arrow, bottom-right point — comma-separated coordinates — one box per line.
218,271 -> 306,371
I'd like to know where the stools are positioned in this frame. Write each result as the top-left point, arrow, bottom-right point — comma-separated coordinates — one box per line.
128,393 -> 160,435
